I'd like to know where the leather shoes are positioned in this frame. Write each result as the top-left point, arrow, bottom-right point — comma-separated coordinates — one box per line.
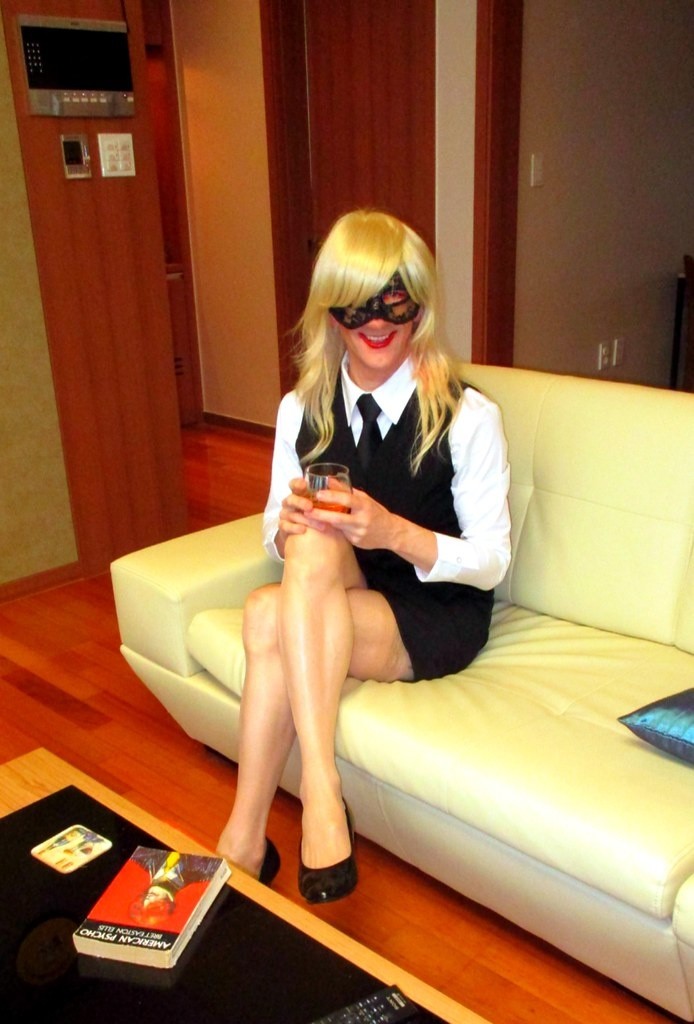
296,796 -> 360,907
258,836 -> 285,883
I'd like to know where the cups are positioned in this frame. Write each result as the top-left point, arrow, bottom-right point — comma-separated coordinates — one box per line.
303,462 -> 350,514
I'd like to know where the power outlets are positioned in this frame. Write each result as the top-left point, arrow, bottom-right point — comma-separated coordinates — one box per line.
599,341 -> 610,371
613,339 -> 624,366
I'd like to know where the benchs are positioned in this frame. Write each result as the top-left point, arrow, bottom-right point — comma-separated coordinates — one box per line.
107,362 -> 694,1024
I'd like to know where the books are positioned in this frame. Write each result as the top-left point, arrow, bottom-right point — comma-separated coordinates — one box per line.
72,846 -> 231,970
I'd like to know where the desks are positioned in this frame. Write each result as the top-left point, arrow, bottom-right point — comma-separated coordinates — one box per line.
0,746 -> 493,1024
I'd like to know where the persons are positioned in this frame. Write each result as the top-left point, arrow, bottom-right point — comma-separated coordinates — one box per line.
214,209 -> 513,905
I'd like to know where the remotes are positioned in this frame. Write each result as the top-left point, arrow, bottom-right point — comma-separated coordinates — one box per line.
309,984 -> 418,1024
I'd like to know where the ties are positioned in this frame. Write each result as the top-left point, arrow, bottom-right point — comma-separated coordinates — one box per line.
355,394 -> 381,472
